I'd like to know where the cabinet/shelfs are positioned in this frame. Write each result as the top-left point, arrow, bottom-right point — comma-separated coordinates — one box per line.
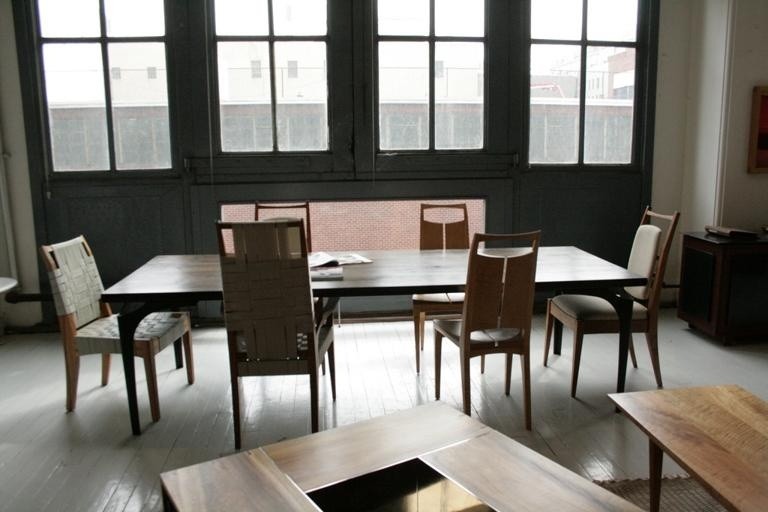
676,231 -> 768,348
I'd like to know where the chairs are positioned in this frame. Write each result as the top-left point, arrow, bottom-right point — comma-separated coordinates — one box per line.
410,202 -> 471,376
253,199 -> 329,379
214,217 -> 338,452
430,230 -> 539,432
542,205 -> 682,400
41,234 -> 196,438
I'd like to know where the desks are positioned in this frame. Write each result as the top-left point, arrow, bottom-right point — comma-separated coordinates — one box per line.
155,399 -> 643,512
606,384 -> 768,512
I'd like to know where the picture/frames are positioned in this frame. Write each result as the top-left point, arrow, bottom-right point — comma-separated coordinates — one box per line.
746,85 -> 768,175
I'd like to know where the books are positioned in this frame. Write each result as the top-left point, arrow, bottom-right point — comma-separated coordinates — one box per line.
308,251 -> 373,269
309,266 -> 344,281
